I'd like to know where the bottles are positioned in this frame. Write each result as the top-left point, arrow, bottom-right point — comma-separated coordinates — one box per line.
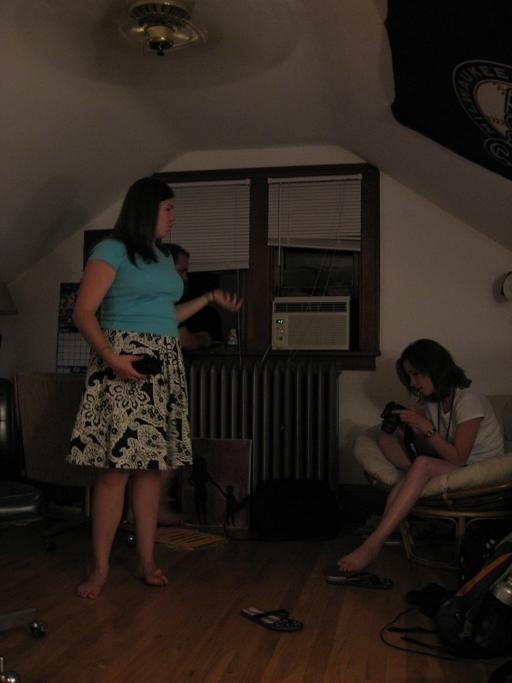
226,327 -> 238,351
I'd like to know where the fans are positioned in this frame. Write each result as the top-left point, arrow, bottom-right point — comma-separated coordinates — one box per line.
18,0 -> 297,94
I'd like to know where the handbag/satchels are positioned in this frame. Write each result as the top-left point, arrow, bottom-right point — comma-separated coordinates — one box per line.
434,534 -> 512,658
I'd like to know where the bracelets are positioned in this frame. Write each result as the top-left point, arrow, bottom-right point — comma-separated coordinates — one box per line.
94,345 -> 114,356
206,289 -> 217,306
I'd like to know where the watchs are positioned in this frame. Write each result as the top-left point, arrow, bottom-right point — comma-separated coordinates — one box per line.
422,425 -> 438,441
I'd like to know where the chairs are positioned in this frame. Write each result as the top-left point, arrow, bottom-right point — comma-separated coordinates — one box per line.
10,364 -> 139,548
380,389 -> 512,574
0,371 -> 54,682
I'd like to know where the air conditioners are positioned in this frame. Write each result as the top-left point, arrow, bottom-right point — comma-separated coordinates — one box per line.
271,293 -> 352,350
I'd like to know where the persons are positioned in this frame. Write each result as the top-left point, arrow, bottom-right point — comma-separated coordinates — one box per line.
186,456 -> 219,527
218,483 -> 238,529
66,172 -> 244,602
337,333 -> 503,572
125,239 -> 210,528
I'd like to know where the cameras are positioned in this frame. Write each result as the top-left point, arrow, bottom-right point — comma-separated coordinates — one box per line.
381,402 -> 408,435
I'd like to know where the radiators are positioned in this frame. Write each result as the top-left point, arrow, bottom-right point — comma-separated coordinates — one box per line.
169,352 -> 342,494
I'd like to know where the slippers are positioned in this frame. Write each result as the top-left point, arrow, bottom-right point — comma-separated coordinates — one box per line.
327,570 -> 393,590
242,606 -> 302,631
